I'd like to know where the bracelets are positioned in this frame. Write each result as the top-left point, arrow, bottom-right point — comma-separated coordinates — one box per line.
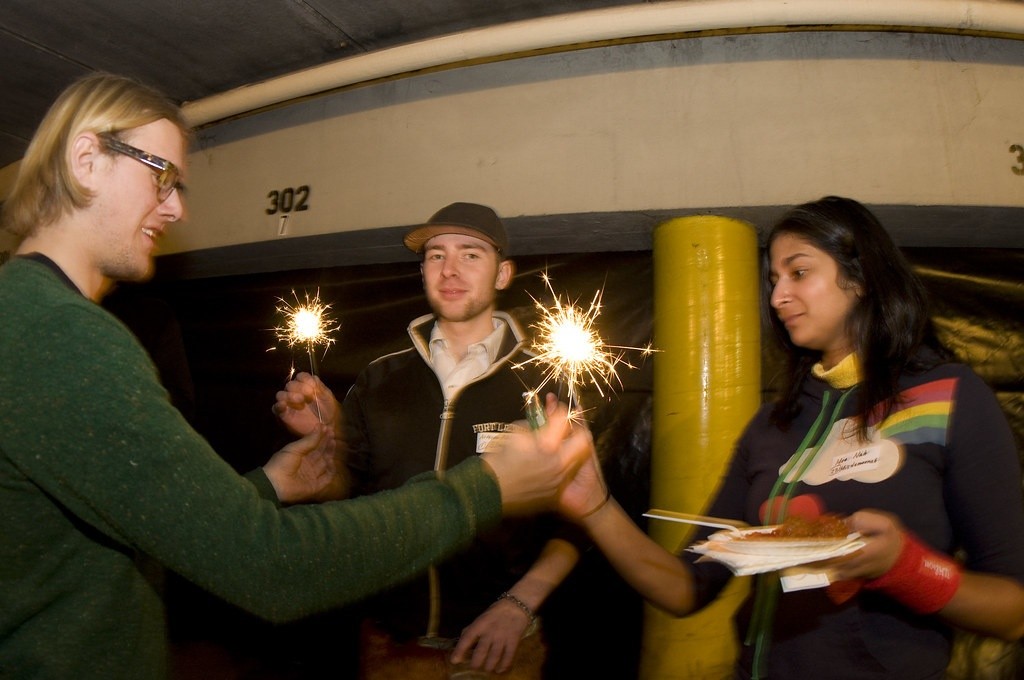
576,491 -> 610,519
498,590 -> 537,639
863,531 -> 963,612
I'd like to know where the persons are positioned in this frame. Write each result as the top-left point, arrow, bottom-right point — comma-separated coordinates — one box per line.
0,74 -> 592,680
273,202 -> 586,680
524,196 -> 1024,680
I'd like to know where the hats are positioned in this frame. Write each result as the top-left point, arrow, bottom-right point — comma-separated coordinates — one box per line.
403,202 -> 508,258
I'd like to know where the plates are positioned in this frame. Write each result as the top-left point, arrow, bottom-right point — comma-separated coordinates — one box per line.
708,523 -> 863,556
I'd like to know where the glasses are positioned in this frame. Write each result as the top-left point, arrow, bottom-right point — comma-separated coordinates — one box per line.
97,135 -> 190,205
